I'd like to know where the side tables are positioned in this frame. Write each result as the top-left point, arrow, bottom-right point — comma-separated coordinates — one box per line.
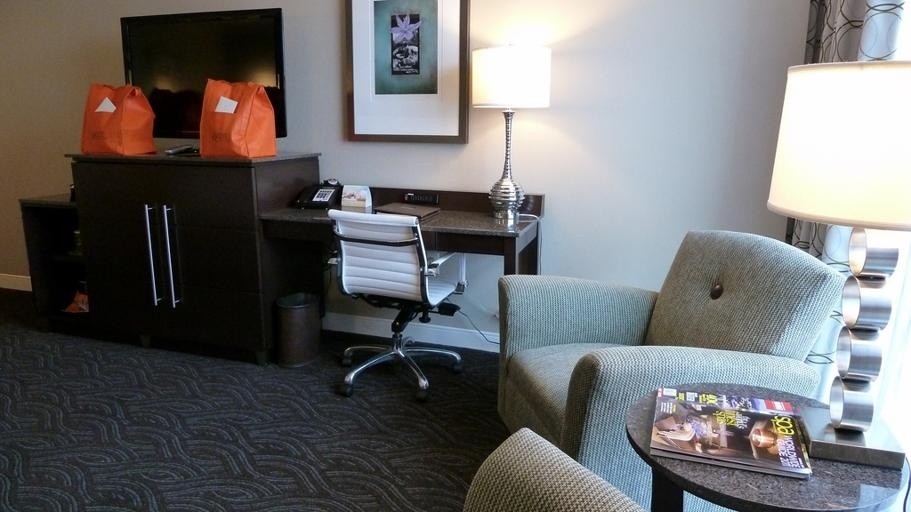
626,382 -> 911,512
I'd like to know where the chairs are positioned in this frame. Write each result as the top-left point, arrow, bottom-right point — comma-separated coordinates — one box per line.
327,207 -> 467,398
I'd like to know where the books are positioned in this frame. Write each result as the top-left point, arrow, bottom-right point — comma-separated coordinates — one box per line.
651,388 -> 813,479
375,203 -> 441,219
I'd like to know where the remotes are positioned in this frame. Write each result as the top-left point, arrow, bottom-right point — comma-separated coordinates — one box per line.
164,144 -> 193,155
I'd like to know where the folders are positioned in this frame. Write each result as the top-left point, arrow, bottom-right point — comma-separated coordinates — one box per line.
374,202 -> 441,225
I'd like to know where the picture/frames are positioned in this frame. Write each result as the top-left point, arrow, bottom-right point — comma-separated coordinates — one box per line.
340,0 -> 474,146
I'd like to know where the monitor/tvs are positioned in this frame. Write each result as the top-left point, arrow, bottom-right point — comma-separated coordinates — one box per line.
120,7 -> 288,157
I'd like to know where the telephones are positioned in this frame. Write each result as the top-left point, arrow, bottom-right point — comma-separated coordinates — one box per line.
295,178 -> 341,210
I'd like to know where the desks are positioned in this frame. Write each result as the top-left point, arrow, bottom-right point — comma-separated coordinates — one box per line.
258,183 -> 546,275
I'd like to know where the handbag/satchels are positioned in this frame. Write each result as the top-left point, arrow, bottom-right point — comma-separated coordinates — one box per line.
78,79 -> 159,162
194,76 -> 279,166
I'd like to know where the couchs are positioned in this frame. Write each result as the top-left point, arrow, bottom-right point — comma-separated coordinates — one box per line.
460,426 -> 653,512
496,230 -> 849,512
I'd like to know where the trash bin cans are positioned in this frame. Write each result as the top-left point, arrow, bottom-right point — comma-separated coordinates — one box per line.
276,292 -> 322,368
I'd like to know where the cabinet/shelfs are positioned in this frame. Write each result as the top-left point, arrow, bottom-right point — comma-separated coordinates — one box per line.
62,151 -> 322,366
16,191 -> 74,337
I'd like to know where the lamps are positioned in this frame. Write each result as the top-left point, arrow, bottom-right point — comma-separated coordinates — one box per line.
470,43 -> 553,220
765,58 -> 910,472
